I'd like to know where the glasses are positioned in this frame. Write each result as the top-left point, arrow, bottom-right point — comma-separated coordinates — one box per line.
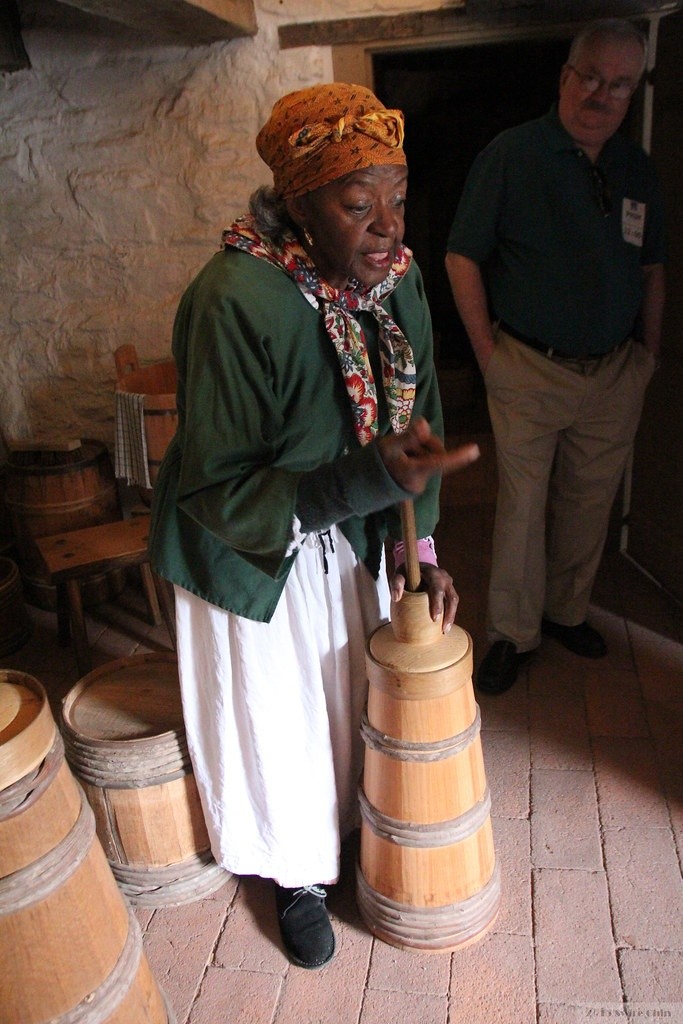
569,65 -> 636,100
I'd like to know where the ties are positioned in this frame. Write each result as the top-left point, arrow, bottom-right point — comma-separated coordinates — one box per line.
220,211 -> 415,447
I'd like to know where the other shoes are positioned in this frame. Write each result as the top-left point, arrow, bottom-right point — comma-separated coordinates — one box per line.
272,882 -> 337,968
340,828 -> 364,882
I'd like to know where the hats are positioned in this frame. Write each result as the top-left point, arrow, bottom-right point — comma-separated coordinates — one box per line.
256,83 -> 409,198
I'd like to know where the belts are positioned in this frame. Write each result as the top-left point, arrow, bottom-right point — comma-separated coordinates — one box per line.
500,319 -> 627,362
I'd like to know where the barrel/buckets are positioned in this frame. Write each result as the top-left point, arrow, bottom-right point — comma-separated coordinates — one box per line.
59,651 -> 234,909
7,433 -> 130,610
113,343 -> 180,510
0,667 -> 177,1024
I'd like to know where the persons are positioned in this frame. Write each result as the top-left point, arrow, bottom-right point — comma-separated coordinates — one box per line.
146,83 -> 481,969
444,18 -> 666,696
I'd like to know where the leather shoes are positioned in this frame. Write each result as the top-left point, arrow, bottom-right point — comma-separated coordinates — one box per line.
540,616 -> 607,658
479,638 -> 540,694
